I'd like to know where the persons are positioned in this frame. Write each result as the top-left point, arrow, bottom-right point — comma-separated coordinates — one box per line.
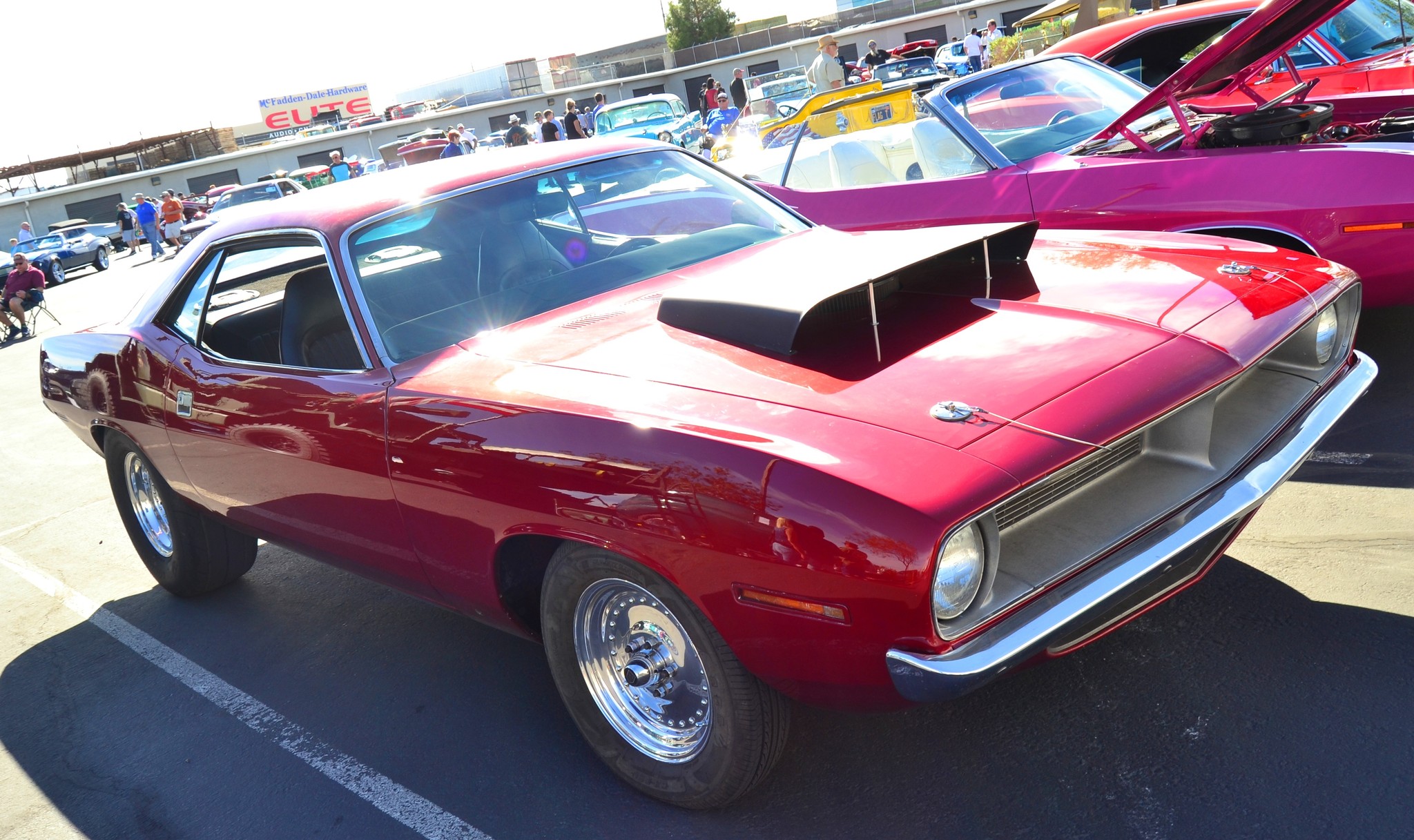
9,238 -> 21,255
980,19 -> 1002,53
131,193 -> 166,259
532,109 -> 568,188
0,252 -> 45,340
116,203 -> 143,256
963,28 -> 984,73
951,36 -> 962,42
328,151 -> 356,184
439,129 -> 463,159
865,40 -> 905,80
446,123 -> 477,152
697,68 -> 806,159
981,47 -> 989,69
19,222 -> 38,251
504,114 -> 533,148
210,185 -> 215,189
807,35 -> 845,93
145,189 -> 199,251
565,92 -> 607,182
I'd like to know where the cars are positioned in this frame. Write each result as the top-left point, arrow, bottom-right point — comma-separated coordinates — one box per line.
1,0 -> 1413,297
38,134 -> 1385,817
542,48 -> 1413,338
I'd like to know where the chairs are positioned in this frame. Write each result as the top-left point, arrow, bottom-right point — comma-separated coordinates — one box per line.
952,61 -> 972,78
476,218 -> 577,332
279,265 -> 426,370
910,120 -> 986,180
829,142 -> 899,190
0,281 -> 61,344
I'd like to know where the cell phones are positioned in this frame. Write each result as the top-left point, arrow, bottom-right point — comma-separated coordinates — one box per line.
10,292 -> 17,297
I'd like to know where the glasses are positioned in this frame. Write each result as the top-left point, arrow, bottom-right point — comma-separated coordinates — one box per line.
987,24 -> 992,27
332,156 -> 339,160
828,44 -> 836,47
15,261 -> 25,266
869,43 -> 874,47
718,100 -> 727,102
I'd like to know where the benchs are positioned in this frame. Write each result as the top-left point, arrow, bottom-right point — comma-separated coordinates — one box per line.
211,247 -> 475,366
755,139 -> 891,191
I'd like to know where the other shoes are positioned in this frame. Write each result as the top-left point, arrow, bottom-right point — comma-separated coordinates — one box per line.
175,244 -> 183,254
128,249 -> 136,256
158,251 -> 166,257
152,254 -> 156,259
6,327 -> 22,339
21,326 -> 30,336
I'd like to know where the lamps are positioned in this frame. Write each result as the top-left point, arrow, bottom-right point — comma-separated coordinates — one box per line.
547,98 -> 554,106
151,177 -> 161,186
968,10 -> 977,19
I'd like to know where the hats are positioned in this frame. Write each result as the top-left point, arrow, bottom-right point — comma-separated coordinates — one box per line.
159,191 -> 169,198
733,68 -> 744,75
715,82 -> 720,85
131,193 -> 146,200
13,252 -> 27,260
168,189 -> 174,194
457,123 -> 465,129
717,92 -> 727,99
817,35 -> 839,51
508,114 -> 520,124
534,111 -> 541,119
116,203 -> 124,207
